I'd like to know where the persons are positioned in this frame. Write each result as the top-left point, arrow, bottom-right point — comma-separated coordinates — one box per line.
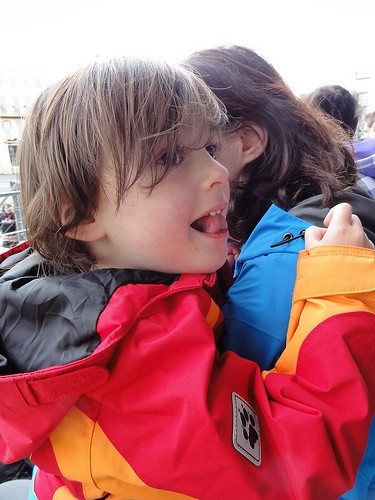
1,204 -> 19,249
301,84 -> 375,186
177,45 -> 375,499
0,59 -> 372,500
